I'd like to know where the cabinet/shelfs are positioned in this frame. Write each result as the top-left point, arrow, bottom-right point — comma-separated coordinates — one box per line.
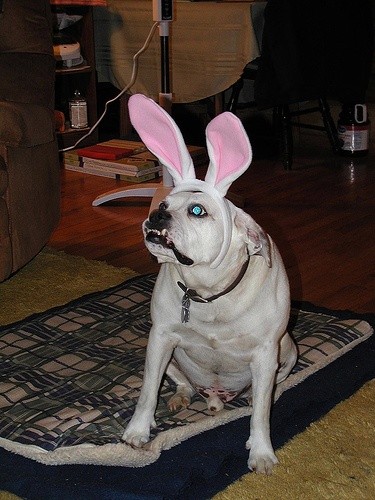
52,4 -> 99,147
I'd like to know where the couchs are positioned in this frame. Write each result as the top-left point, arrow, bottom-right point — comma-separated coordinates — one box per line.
0,0 -> 62,282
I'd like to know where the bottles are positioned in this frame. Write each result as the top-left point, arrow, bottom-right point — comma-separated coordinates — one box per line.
68,90 -> 88,129
336,104 -> 369,157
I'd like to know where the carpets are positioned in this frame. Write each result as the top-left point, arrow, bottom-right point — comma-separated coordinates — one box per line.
1,247 -> 375,500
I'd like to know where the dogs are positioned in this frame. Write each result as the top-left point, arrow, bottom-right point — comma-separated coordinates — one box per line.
120,192 -> 298,474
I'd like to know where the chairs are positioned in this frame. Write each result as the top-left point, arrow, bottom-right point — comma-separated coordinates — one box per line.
226,0 -> 341,172
